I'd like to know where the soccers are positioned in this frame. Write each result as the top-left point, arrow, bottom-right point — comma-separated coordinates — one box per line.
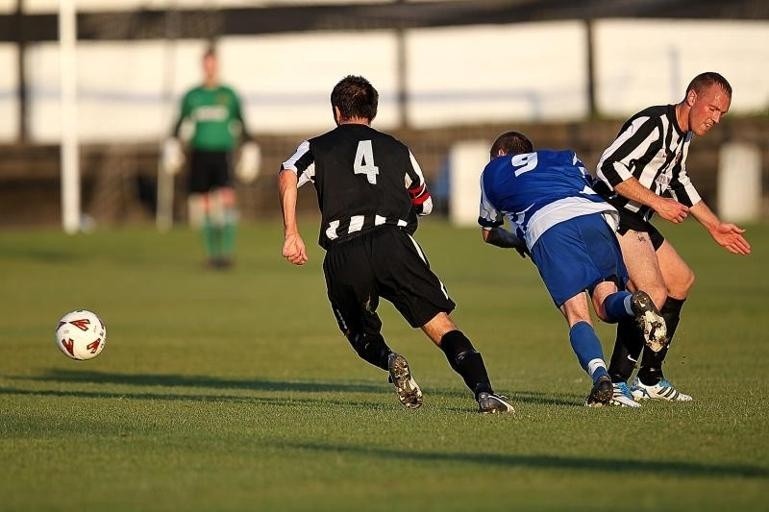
56,307 -> 107,362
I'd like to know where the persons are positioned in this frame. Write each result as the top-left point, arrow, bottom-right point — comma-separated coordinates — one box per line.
275,76 -> 514,415
163,46 -> 264,277
596,71 -> 754,406
474,127 -> 668,413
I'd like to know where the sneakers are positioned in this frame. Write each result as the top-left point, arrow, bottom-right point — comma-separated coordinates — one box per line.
629,289 -> 668,353
610,382 -> 643,409
630,376 -> 692,402
588,378 -> 614,406
385,347 -> 424,410
477,391 -> 516,414
